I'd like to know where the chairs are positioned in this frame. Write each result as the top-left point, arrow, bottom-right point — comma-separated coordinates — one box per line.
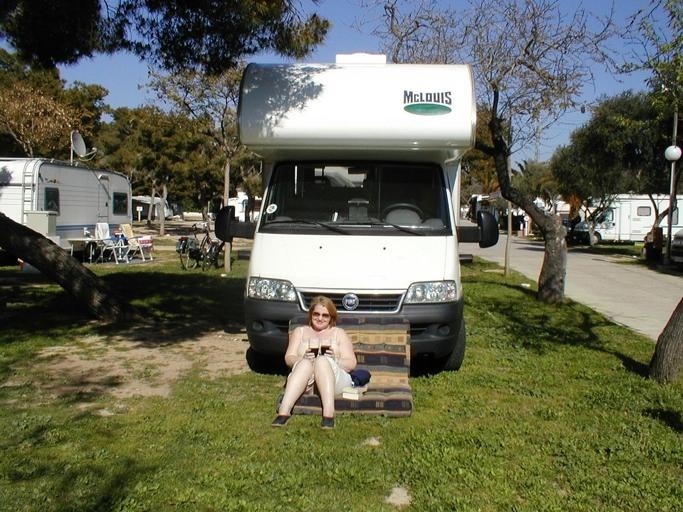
95,222 -> 153,264
275,316 -> 414,416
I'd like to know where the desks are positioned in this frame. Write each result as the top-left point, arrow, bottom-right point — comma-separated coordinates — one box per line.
67,239 -> 104,266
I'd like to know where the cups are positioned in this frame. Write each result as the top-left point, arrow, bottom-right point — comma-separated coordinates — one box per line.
309,336 -> 319,358
321,338 -> 331,356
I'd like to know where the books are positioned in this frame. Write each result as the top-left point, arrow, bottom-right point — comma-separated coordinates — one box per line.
341,388 -> 363,400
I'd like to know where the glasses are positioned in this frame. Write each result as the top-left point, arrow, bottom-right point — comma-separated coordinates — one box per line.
313,312 -> 329,318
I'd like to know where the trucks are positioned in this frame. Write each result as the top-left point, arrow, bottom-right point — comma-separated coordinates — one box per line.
214,52 -> 500,374
570,193 -> 683,245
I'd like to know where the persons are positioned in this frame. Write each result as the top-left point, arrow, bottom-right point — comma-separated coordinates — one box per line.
270,295 -> 357,430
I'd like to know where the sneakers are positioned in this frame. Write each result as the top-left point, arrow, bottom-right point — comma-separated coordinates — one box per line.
271,414 -> 291,426
320,416 -> 334,429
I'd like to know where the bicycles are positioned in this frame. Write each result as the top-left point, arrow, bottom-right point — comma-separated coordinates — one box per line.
179,223 -> 221,270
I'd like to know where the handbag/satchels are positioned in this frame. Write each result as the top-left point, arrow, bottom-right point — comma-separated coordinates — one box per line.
350,369 -> 371,387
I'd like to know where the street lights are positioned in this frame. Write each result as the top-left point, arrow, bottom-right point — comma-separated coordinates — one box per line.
661,83 -> 683,266
